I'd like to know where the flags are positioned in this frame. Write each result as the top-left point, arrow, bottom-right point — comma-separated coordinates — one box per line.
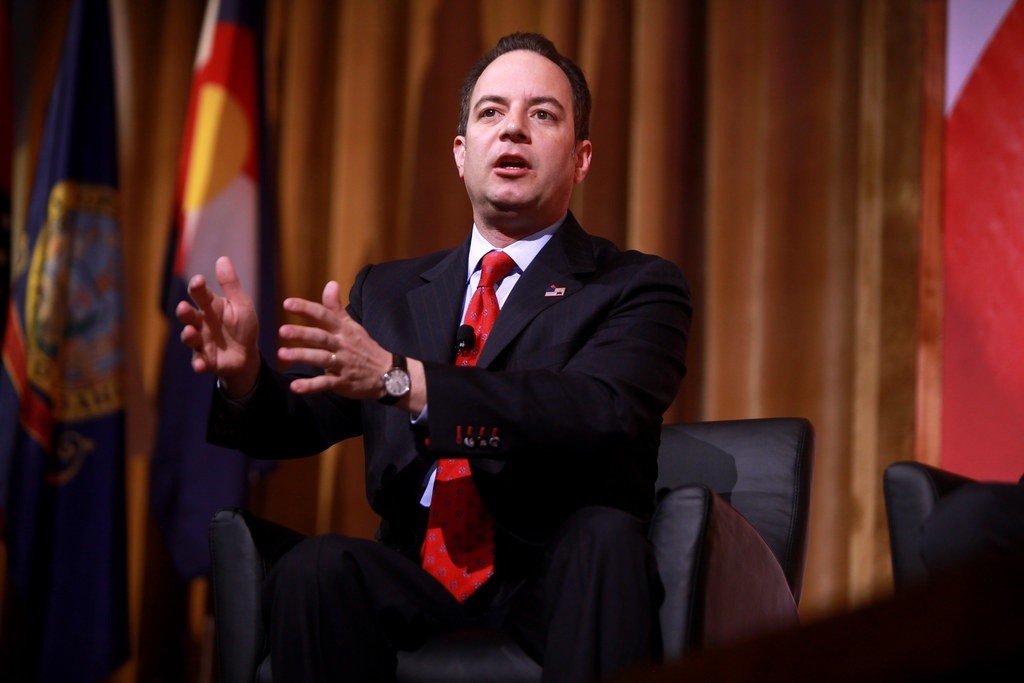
0,1 -> 259,683
941,0 -> 1023,486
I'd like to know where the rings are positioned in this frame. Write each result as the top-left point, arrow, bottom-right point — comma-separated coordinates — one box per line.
330,353 -> 337,371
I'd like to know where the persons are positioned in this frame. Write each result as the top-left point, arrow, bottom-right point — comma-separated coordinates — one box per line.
177,32 -> 697,683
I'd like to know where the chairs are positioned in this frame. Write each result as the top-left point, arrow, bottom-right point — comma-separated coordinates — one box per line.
208,418 -> 814,683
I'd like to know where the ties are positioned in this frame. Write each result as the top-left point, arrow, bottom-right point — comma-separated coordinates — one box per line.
419,250 -> 517,607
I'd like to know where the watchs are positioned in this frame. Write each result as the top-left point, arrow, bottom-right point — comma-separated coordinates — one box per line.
379,351 -> 413,407
